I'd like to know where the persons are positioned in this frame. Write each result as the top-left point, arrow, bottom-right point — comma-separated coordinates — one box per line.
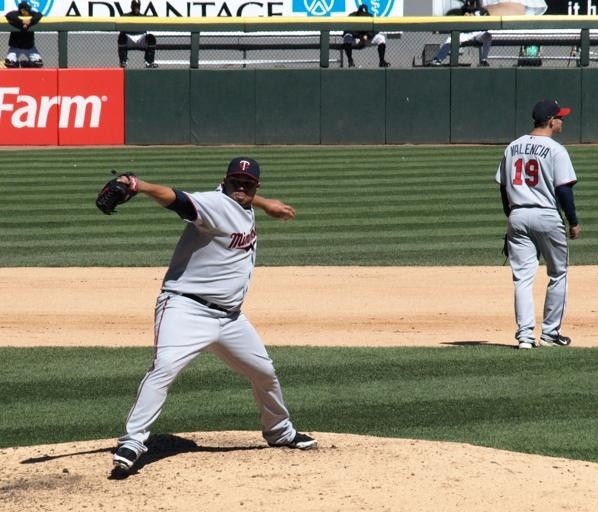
430,0 -> 492,66
5,1 -> 43,68
112,157 -> 318,471
342,4 -> 391,67
494,100 -> 580,349
117,0 -> 159,68
485,3 -> 526,16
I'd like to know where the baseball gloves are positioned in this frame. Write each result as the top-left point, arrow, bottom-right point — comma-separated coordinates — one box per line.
96,170 -> 137,216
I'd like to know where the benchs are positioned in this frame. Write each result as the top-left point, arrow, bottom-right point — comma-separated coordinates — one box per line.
475,35 -> 598,64
129,43 -> 346,68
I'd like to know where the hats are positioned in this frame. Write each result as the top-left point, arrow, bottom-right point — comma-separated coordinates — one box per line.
532,99 -> 571,121
18,1 -> 31,10
131,0 -> 141,8
359,4 -> 368,16
227,157 -> 260,182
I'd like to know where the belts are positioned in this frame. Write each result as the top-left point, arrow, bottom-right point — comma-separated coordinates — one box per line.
161,290 -> 231,314
511,204 -> 548,210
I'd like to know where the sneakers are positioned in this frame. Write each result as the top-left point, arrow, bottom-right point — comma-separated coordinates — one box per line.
538,334 -> 570,347
425,59 -> 440,67
348,61 -> 354,67
286,431 -> 318,450
112,443 -> 142,471
518,341 -> 535,349
480,61 -> 489,66
144,61 -> 158,68
120,60 -> 126,66
379,61 -> 390,67
